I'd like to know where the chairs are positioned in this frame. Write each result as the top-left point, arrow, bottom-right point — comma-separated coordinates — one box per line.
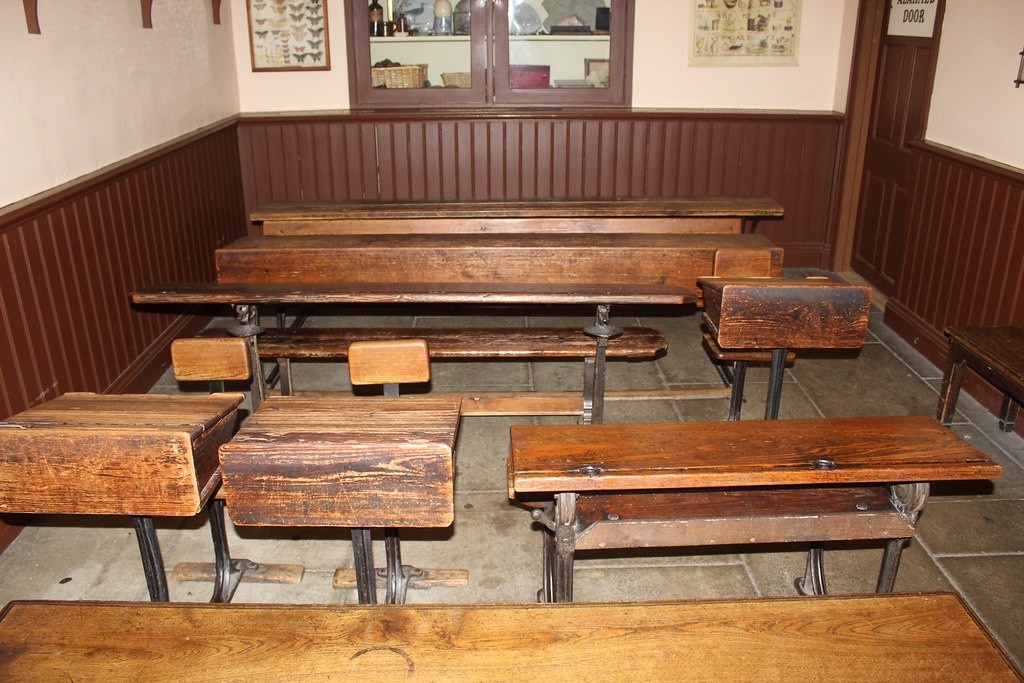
170,338 -> 306,607
333,339 -> 470,607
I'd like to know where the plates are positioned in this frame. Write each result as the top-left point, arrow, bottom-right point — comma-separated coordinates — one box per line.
393,32 -> 410,37
414,31 -> 432,36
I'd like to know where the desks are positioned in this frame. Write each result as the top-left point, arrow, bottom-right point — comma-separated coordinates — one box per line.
507,415 -> 1004,601
248,195 -> 785,241
0,591 -> 1023,683
216,231 -> 785,307
131,284 -> 698,428
217,395 -> 465,605
0,392 -> 247,600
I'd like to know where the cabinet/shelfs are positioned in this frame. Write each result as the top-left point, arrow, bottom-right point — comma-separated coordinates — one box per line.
353,0 -> 623,107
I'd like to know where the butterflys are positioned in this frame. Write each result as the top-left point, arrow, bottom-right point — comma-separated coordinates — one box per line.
253,0 -> 324,66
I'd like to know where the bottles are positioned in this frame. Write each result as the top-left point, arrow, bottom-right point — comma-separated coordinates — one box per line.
397,13 -> 407,32
432,0 -> 452,36
368,0 -> 383,35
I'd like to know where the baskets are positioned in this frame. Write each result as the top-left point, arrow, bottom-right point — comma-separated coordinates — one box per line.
369,63 -> 429,90
441,72 -> 471,89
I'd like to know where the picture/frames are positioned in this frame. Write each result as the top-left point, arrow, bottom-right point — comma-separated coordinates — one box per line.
246,0 -> 330,72
584,58 -> 609,80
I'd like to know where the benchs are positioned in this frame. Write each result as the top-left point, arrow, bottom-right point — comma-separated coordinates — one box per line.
196,328 -> 669,415
936,324 -> 1024,434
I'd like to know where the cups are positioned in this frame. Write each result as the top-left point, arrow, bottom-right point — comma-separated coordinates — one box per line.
382,22 -> 392,36
453,12 -> 472,35
